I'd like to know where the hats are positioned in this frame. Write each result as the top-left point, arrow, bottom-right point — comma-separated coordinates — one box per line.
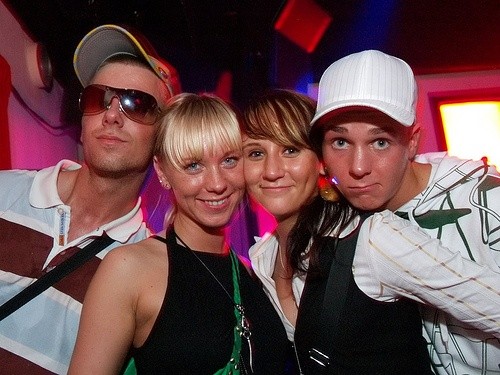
74,25 -> 182,98
309,49 -> 419,127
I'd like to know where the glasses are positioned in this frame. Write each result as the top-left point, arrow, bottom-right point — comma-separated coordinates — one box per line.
79,84 -> 164,126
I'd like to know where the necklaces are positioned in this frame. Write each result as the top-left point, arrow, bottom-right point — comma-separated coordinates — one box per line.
172,223 -> 251,339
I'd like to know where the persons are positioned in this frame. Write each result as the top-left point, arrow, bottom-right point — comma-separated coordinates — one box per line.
67,92 -> 301,375
0,25 -> 181,375
310,49 -> 500,375
238,89 -> 437,375
